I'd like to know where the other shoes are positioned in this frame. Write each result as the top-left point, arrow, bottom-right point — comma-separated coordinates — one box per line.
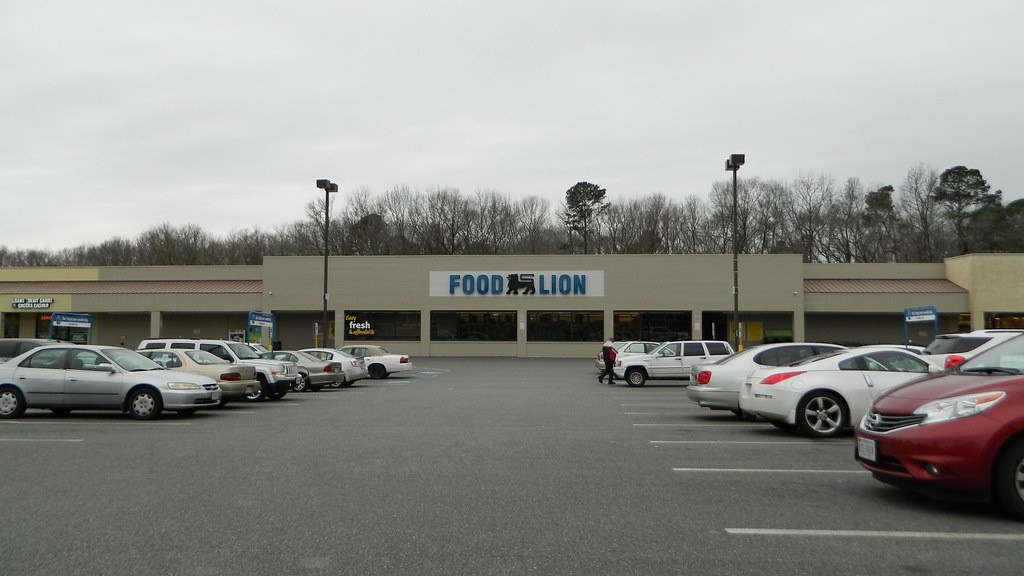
597,376 -> 616,384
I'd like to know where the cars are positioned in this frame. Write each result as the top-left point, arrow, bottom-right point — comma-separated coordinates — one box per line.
686,342 -> 849,419
0,345 -> 224,421
739,346 -> 943,438
262,351 -> 344,392
596,341 -> 675,373
0,338 -> 75,363
339,345 -> 413,378
134,349 -> 261,409
299,348 -> 369,388
854,332 -> 1024,520
643,326 -> 689,340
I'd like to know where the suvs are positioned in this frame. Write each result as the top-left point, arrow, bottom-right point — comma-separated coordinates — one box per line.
613,341 -> 734,386
918,334 -> 1023,371
138,338 -> 299,401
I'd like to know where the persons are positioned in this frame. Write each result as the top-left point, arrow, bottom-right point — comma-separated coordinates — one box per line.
597,337 -> 619,386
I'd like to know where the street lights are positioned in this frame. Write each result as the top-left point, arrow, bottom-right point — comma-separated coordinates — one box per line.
316,179 -> 338,347
725,153 -> 746,352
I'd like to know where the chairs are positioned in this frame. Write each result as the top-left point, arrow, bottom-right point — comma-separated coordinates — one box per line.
797,350 -> 909,372
34,353 -> 182,377
